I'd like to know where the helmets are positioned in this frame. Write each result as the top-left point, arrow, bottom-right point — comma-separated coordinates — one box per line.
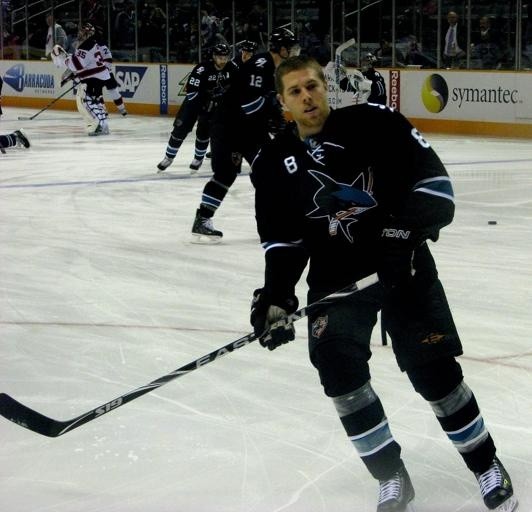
214,44 -> 229,56
81,23 -> 95,38
360,52 -> 376,72
270,27 -> 296,53
241,40 -> 257,53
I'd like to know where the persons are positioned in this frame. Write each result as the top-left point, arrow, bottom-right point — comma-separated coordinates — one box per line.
249,55 -> 513,512
0,0 -> 507,170
192,27 -> 302,236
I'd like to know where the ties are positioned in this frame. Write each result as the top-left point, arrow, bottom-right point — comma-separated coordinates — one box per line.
447,28 -> 454,52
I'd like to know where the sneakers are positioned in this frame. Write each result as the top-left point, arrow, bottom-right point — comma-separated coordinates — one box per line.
192,218 -> 223,236
190,159 -> 202,170
15,131 -> 30,148
157,157 -> 173,170
88,124 -> 110,136
377,464 -> 415,511
121,110 -> 128,116
473,456 -> 513,510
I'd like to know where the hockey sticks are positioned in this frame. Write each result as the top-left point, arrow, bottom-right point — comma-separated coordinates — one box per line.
1,265 -> 383,437
18,80 -> 79,120
333,38 -> 356,109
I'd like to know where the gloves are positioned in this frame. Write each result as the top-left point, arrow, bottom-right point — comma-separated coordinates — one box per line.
250,289 -> 299,351
376,248 -> 416,284
